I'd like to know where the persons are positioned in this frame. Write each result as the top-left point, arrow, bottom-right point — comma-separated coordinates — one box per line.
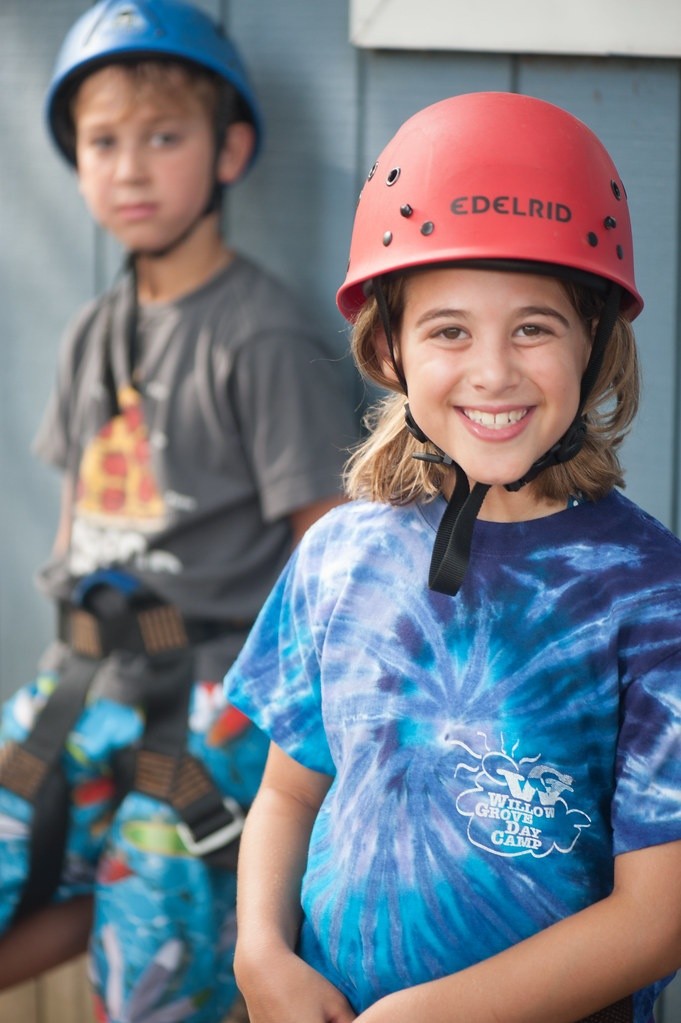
1,0 -> 681,1023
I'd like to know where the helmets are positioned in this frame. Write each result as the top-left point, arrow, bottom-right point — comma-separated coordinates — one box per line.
335,91 -> 644,323
45,0 -> 263,184
